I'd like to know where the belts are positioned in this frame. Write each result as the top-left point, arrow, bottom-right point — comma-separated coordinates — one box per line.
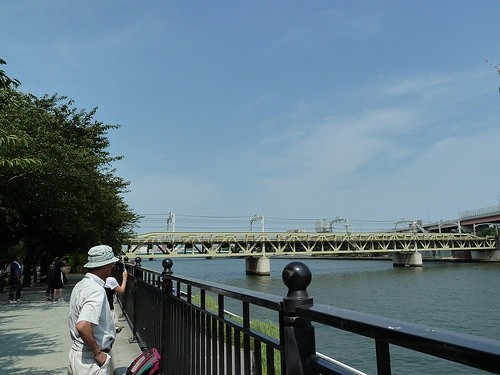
101,348 -> 110,353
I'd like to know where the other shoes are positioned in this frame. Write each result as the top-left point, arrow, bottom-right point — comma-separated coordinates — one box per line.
48,298 -> 64,304
17,299 -> 23,304
116,329 -> 121,333
8,300 -> 17,303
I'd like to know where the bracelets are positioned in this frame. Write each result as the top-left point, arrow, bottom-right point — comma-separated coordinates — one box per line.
123,277 -> 127,281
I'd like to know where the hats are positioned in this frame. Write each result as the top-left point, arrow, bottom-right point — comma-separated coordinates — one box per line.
83,245 -> 120,268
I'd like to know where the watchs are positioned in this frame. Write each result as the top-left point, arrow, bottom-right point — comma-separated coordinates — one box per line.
95,350 -> 102,356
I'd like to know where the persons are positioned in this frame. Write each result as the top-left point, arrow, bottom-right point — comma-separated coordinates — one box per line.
68,245 -> 127,375
9,254 -> 22,303
46,262 -> 68,301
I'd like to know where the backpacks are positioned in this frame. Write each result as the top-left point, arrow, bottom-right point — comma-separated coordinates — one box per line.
126,347 -> 161,375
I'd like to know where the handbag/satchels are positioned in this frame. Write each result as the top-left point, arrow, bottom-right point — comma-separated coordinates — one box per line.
61,272 -> 64,285
